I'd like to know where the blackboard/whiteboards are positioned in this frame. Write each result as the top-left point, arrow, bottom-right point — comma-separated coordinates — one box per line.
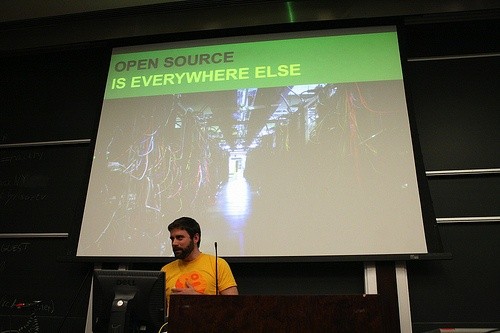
0,0 -> 499,330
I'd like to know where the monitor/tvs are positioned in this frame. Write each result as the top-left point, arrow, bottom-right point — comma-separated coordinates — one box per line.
92,269 -> 166,333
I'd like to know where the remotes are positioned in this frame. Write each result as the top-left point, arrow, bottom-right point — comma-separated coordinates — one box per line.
214,242 -> 220,295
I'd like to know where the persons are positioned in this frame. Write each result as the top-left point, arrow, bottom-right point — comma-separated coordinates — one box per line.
158,216 -> 241,299
